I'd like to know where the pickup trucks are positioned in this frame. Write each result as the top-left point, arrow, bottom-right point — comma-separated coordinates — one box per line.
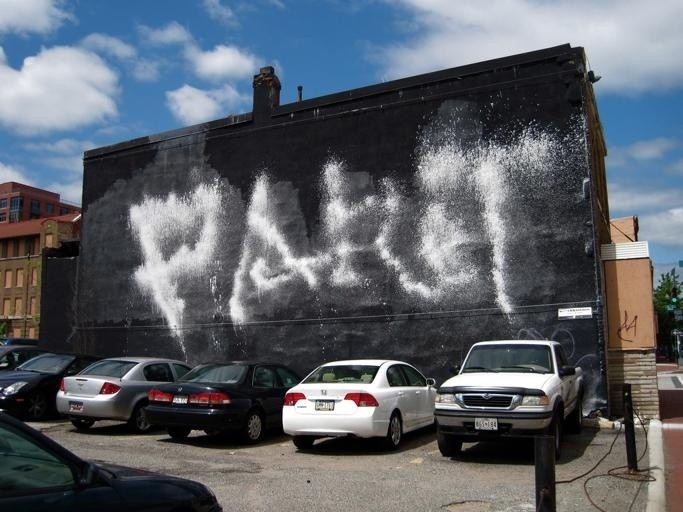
433,339 -> 584,462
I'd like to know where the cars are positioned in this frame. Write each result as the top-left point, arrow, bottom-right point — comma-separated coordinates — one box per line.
0,352 -> 102,422
55,355 -> 193,434
143,360 -> 300,445
281,358 -> 437,452
0,344 -> 37,369
0,407 -> 221,512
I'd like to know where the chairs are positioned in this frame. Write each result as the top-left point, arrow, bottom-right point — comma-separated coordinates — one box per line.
321,371 -> 372,383
